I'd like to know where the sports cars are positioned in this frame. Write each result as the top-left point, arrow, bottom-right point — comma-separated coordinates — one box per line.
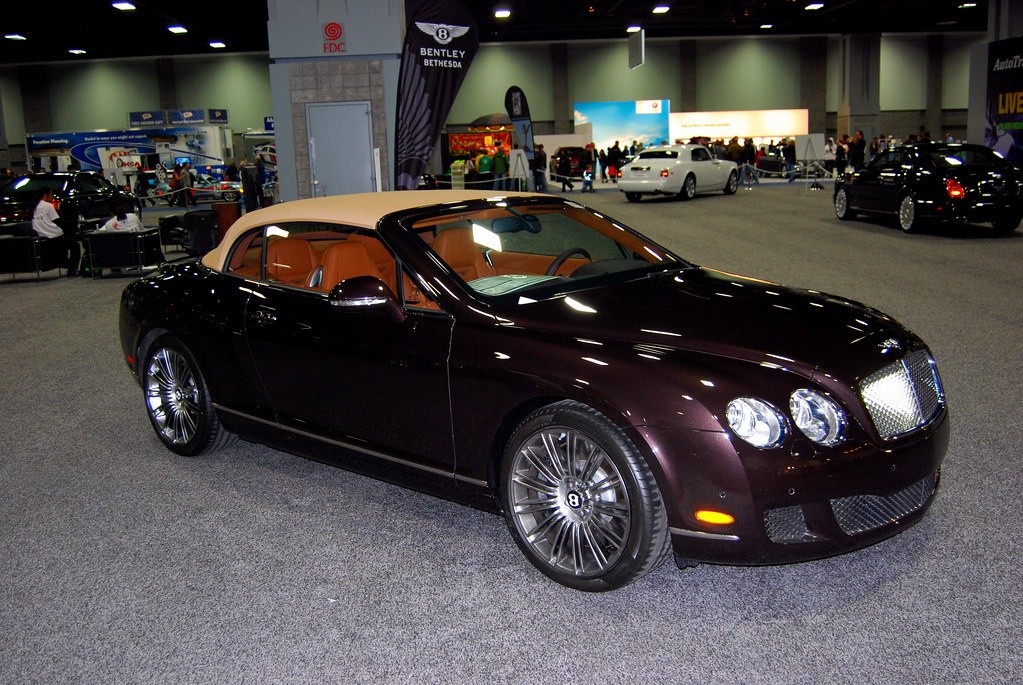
115,186 -> 953,595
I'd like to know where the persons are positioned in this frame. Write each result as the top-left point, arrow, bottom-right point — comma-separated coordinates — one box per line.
581,165 -> 596,193
586,141 -> 642,184
134,166 -> 156,208
825,130 -> 878,176
945,133 -> 953,143
902,131 -> 931,145
464,146 -> 509,191
168,162 -> 199,208
556,149 -> 574,192
32,187 -> 81,277
712,136 -> 796,182
224,159 -> 246,182
534,142 -> 548,192
101,200 -> 144,232
662,140 -> 684,144
0,167 -> 14,182
254,155 -> 266,199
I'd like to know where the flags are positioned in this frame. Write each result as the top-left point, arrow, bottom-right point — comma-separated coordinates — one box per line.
394,0 -> 479,191
504,86 -> 534,171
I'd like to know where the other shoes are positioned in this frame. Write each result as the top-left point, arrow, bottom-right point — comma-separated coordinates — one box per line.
562,190 -> 566,192
570,186 -> 573,192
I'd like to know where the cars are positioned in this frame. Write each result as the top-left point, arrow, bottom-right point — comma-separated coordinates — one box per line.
616,143 -> 740,204
252,143 -> 277,166
0,167 -> 143,269
549,146 -> 593,183
154,172 -> 244,206
831,141 -> 1023,237
757,143 -> 823,179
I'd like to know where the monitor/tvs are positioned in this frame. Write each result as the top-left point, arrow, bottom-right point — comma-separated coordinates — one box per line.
175,157 -> 191,167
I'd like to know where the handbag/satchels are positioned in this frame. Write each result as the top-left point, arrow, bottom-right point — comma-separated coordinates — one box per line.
169,180 -> 176,188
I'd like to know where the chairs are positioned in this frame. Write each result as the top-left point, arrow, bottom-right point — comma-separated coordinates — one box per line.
264,227 -> 496,310
78,210 -> 218,281
0,222 -> 68,280
